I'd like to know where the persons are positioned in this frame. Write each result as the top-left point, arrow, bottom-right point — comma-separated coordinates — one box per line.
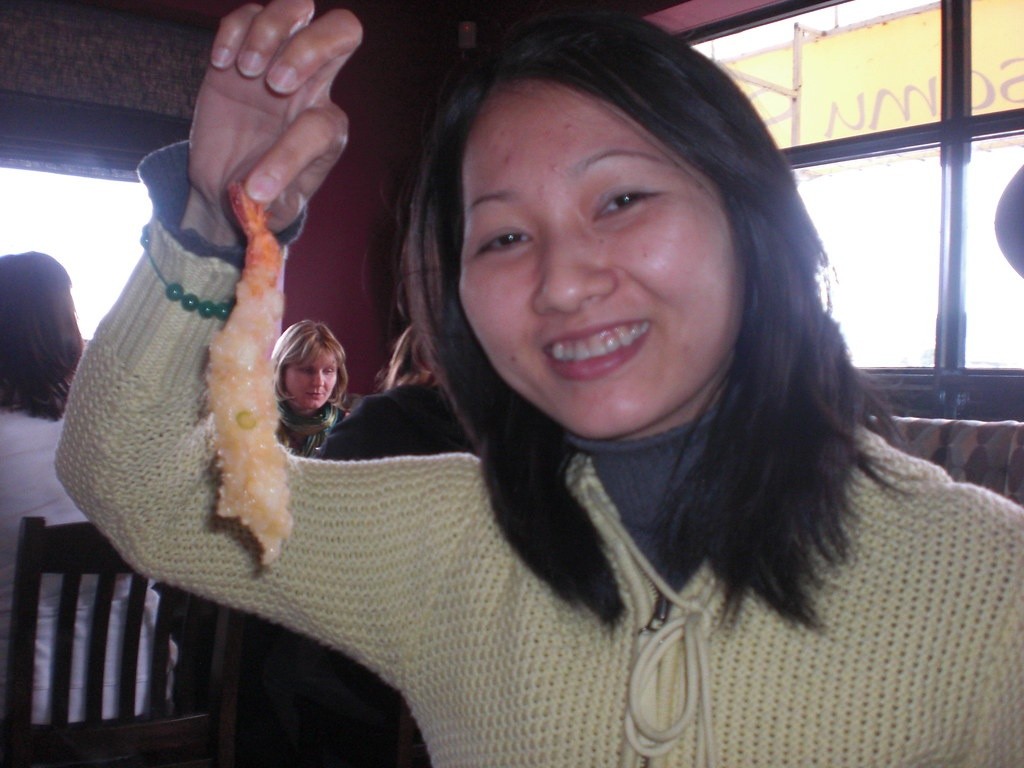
54,0 -> 1024,768
0,252 -> 472,768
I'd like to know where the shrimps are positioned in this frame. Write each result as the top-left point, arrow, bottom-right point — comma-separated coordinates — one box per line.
201,177 -> 297,565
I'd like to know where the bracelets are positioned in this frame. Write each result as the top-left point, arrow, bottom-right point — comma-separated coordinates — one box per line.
142,225 -> 238,320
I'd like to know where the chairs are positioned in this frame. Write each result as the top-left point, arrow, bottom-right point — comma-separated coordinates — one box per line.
2,517 -> 243,768
290,675 -> 432,768
